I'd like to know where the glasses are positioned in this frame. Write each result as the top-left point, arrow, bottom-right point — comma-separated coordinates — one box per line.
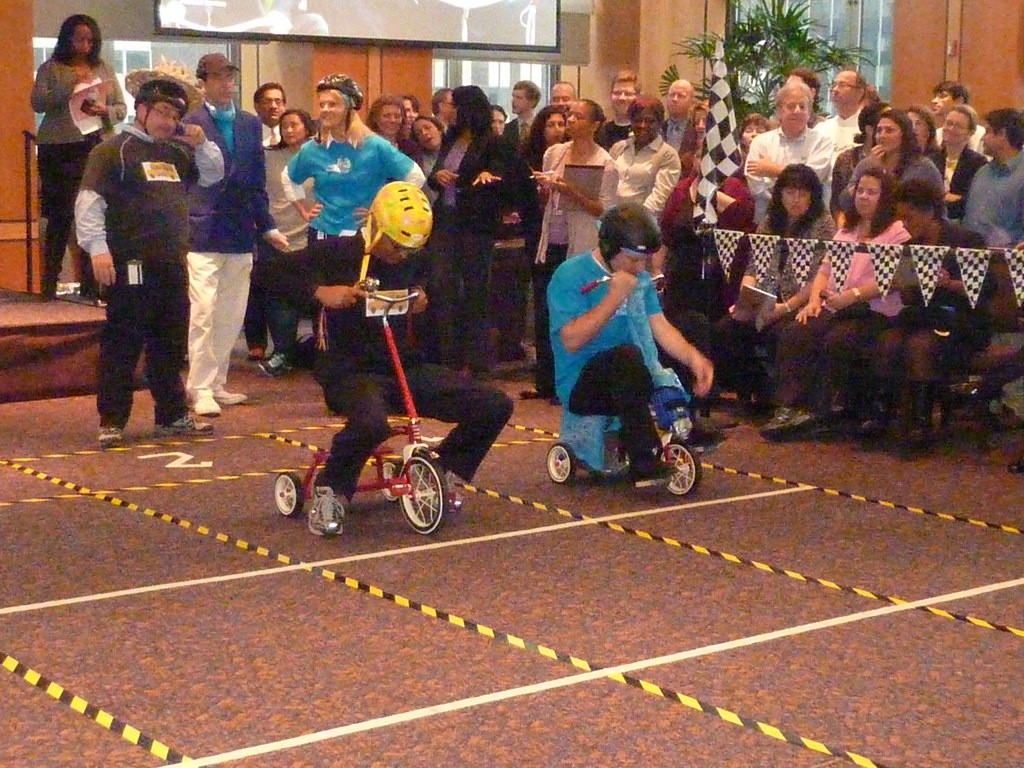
386,235 -> 420,253
149,105 -> 179,122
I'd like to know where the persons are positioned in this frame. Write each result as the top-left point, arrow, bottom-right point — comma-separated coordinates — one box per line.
431,88 -> 454,126
497,68 -> 1024,474
426,85 -> 513,375
489,104 -> 508,136
280,72 -> 425,372
545,202 -> 726,488
411,115 -> 446,178
257,109 -> 316,377
290,181 -> 514,537
401,94 -> 420,139
74,64 -> 224,445
365,95 -> 423,171
31,14 -> 127,300
182,53 -> 289,417
253,82 -> 287,147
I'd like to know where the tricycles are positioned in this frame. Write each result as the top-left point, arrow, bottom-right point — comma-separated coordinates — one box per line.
273,278 -> 464,534
546,274 -> 703,497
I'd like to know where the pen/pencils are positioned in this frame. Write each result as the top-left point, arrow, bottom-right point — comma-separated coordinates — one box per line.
529,171 -> 554,179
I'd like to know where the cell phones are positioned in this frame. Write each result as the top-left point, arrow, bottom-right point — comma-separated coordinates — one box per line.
176,123 -> 184,135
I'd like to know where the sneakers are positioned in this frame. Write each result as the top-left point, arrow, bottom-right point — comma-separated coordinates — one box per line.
310,485 -> 344,535
258,352 -> 293,376
97,425 -> 124,449
155,415 -> 215,436
431,451 -> 462,511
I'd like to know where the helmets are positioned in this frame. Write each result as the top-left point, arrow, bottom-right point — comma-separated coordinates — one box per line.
598,202 -> 662,261
370,180 -> 434,248
316,71 -> 363,111
134,81 -> 188,121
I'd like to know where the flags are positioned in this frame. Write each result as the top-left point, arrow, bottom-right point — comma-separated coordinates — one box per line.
691,40 -> 745,236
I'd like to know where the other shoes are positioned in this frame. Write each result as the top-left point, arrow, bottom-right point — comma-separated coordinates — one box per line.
518,388 -> 546,399
629,462 -> 679,481
211,389 -> 247,406
550,397 -> 562,406
491,343 -> 526,361
684,427 -> 724,451
248,344 -> 265,361
735,379 -> 1024,471
184,391 -> 221,415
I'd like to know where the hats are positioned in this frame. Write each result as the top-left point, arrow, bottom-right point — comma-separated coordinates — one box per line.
195,52 -> 241,78
124,70 -> 205,112
